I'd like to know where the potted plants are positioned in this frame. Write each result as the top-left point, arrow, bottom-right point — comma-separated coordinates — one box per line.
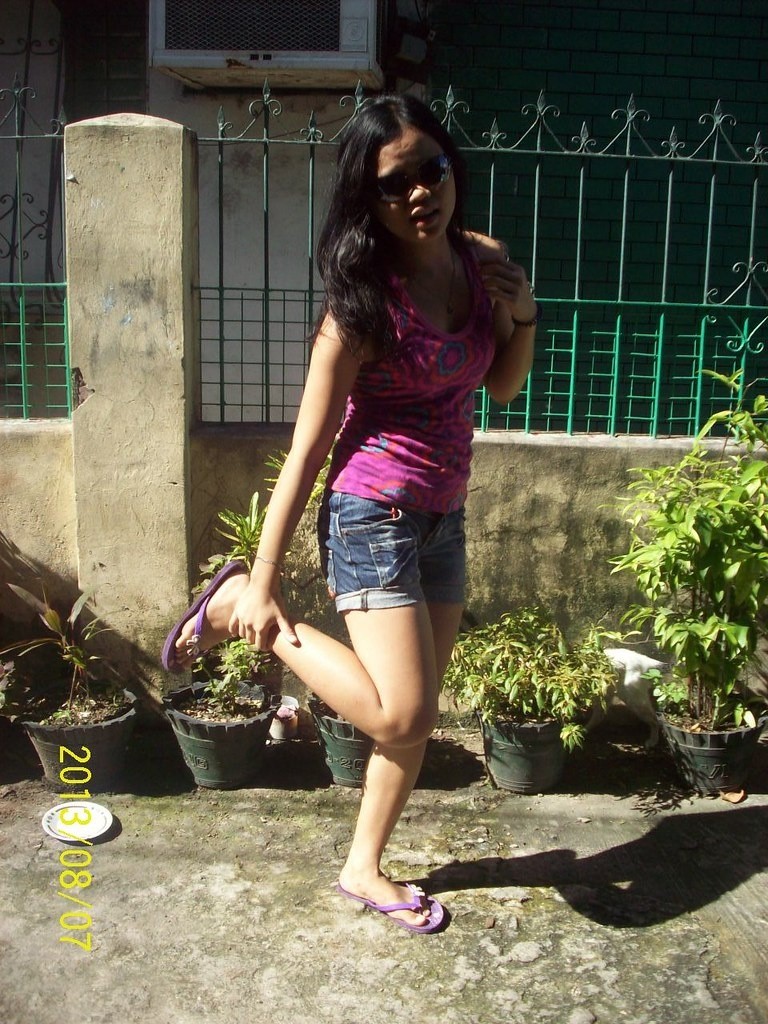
1,360 -> 767,796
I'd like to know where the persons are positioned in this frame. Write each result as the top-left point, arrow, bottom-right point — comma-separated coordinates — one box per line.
159,95 -> 537,935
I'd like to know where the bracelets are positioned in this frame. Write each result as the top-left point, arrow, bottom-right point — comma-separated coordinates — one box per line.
511,302 -> 541,327
255,557 -> 278,567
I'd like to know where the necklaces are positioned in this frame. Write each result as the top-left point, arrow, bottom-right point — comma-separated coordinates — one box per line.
412,246 -> 455,314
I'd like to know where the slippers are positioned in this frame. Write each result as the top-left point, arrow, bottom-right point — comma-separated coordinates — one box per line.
336,876 -> 445,934
161,559 -> 245,674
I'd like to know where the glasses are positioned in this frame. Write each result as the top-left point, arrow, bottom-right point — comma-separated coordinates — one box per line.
378,151 -> 457,202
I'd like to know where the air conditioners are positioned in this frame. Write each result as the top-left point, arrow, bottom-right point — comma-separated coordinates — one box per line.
146,0 -> 389,90
